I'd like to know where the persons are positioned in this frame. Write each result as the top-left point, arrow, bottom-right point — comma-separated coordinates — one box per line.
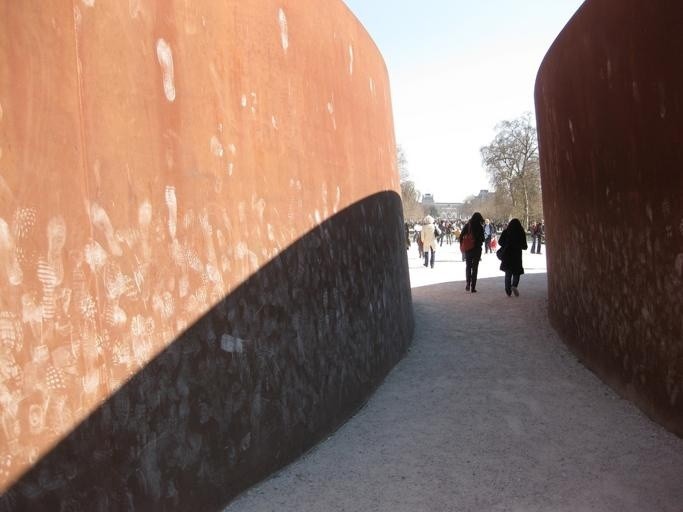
405,215 -> 544,268
496,218 -> 528,298
460,212 -> 486,293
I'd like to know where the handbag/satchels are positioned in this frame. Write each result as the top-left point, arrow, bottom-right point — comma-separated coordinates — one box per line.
496,246 -> 508,261
435,229 -> 440,238
460,234 -> 474,252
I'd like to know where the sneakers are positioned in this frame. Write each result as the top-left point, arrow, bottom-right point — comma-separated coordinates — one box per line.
466,287 -> 470,291
512,287 -> 520,296
471,289 -> 477,293
